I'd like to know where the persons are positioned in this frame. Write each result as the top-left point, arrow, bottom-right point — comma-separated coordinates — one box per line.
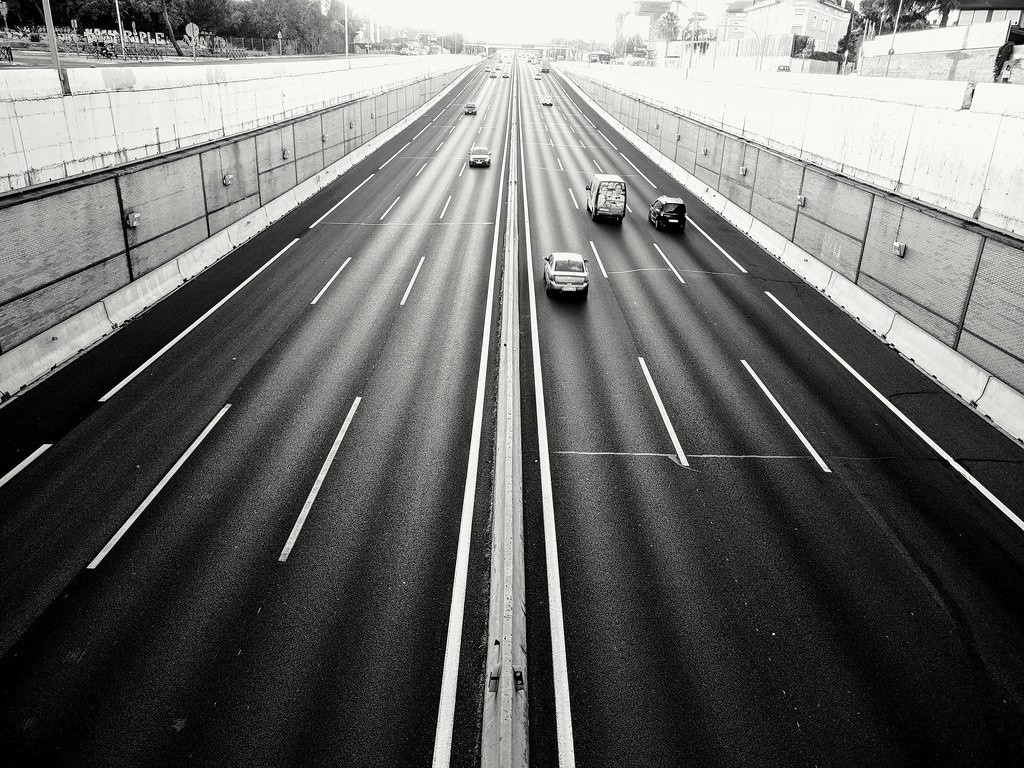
95,40 -> 118,60
1000,65 -> 1011,84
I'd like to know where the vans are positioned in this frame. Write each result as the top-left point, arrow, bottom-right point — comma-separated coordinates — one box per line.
586,174 -> 626,224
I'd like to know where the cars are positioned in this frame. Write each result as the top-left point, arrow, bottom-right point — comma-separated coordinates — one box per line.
482,50 -> 550,81
541,96 -> 554,106
399,47 -> 411,55
464,103 -> 478,115
542,252 -> 590,298
468,147 -> 492,167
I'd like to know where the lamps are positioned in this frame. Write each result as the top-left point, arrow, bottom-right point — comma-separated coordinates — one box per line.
892,241 -> 906,256
675,135 -> 680,141
127,213 -> 140,227
350,121 -> 355,128
796,196 -> 805,206
739,167 -> 745,175
283,150 -> 291,159
654,122 -> 660,130
221,175 -> 234,185
702,148 -> 706,155
323,134 -> 329,142
371,113 -> 375,119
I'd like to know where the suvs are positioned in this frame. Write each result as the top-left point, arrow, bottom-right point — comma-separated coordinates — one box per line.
648,195 -> 687,234
